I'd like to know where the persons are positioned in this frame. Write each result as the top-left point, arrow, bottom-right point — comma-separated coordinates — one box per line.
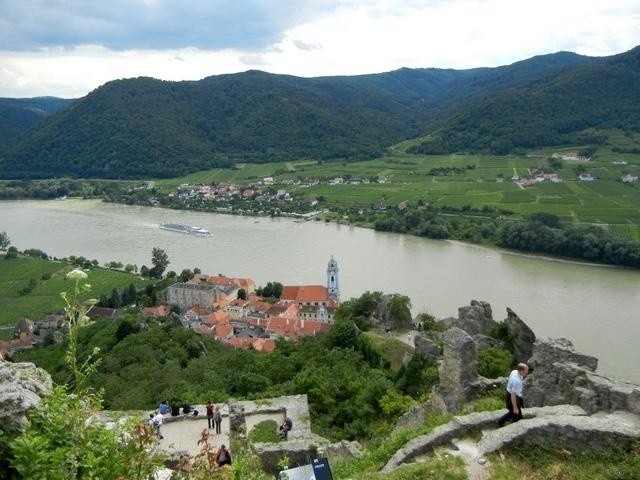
205,401 -> 215,429
169,401 -> 179,416
182,400 -> 196,414
494,362 -> 530,431
153,409 -> 166,439
215,444 -> 231,467
148,413 -> 159,439
213,405 -> 223,434
159,400 -> 169,414
278,416 -> 293,439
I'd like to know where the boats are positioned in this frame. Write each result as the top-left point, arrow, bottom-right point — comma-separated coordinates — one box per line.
158,221 -> 213,238
